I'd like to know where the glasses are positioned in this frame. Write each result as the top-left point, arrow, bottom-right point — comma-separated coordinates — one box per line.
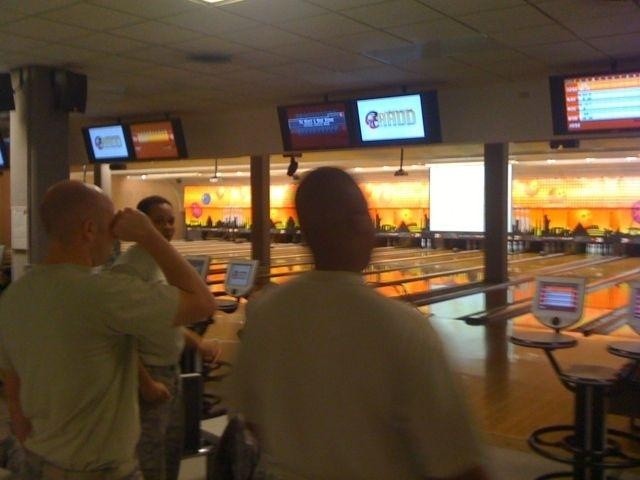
151,217 -> 175,228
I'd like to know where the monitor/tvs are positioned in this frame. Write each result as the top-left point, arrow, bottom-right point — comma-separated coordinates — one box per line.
548,65 -> 640,135
351,88 -> 443,148
275,98 -> 349,151
126,117 -> 188,163
80,122 -> 134,164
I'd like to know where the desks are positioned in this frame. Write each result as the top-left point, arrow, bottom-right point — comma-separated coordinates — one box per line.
508,329 -> 640,480
179,298 -> 239,458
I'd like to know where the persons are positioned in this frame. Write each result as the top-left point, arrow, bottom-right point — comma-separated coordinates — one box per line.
107,193 -> 224,480
226,165 -> 490,479
1,179 -> 219,480
